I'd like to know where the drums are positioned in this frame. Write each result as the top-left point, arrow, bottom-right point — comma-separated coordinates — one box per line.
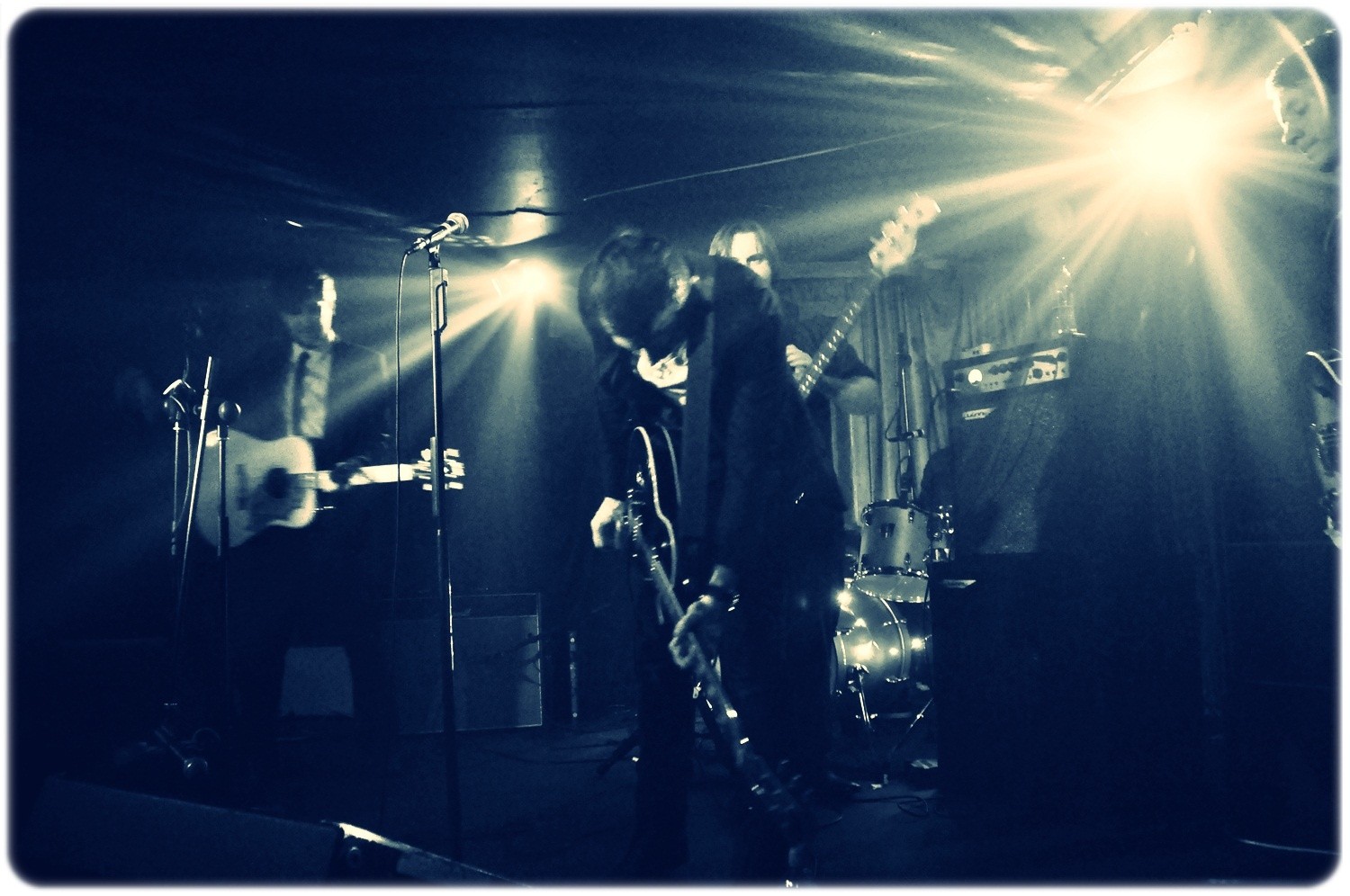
854,495 -> 956,603
829,578 -> 912,730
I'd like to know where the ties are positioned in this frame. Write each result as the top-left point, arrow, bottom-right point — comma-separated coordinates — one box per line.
292,351 -> 311,437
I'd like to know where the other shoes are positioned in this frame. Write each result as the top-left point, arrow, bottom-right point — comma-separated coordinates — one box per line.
742,830 -> 811,878
610,824 -> 691,881
802,769 -> 873,802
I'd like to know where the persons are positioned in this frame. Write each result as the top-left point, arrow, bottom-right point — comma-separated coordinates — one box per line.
205,267 -> 411,817
575,219 -> 885,883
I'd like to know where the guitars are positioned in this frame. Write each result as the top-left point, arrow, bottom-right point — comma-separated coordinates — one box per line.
795,190 -> 942,401
625,420 -> 814,820
188,426 -> 466,549
